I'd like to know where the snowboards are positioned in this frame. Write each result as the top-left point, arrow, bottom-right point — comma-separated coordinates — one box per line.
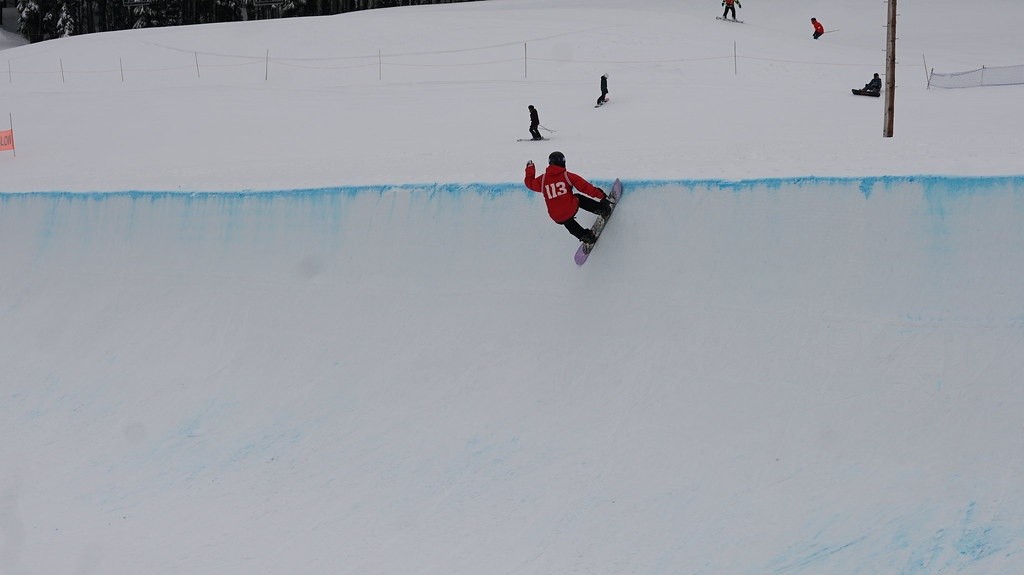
573,177 -> 622,266
851,89 -> 881,97
595,97 -> 609,107
716,16 -> 743,23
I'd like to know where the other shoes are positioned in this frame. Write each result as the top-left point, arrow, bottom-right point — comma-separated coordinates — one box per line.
531,137 -> 536,140
536,137 -> 541,140
579,228 -> 594,244
600,200 -> 611,215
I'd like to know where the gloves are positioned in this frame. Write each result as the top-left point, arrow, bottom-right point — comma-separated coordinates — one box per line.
722,3 -> 724,6
604,195 -> 616,204
739,5 -> 741,8
526,160 -> 534,167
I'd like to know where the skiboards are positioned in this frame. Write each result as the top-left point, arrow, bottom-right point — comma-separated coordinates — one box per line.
517,138 -> 550,142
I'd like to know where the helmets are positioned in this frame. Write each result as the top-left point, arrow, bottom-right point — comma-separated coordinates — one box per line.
604,73 -> 609,78
528,105 -> 534,110
811,18 -> 816,23
874,73 -> 879,79
548,152 -> 565,166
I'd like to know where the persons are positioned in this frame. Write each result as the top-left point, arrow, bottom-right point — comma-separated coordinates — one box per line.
722,0 -> 741,20
862,73 -> 882,93
811,18 -> 824,39
528,105 -> 542,141
524,151 -> 612,245
597,73 -> 609,105
